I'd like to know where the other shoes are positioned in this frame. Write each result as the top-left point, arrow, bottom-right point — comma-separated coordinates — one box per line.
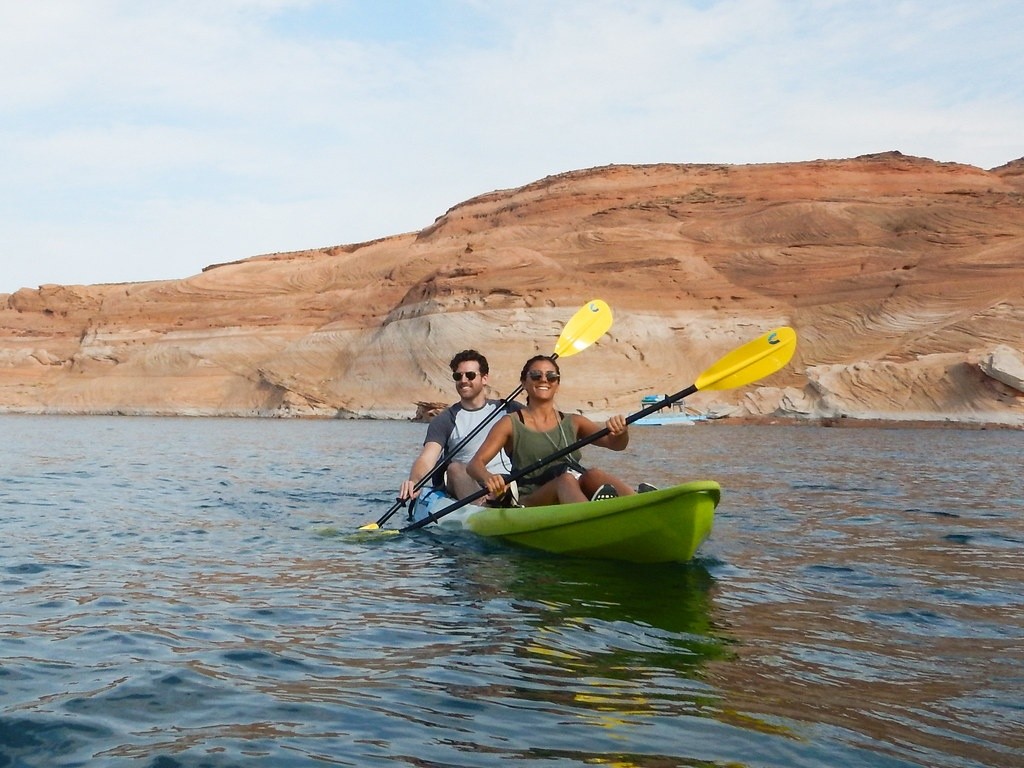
590,484 -> 619,501
638,482 -> 657,493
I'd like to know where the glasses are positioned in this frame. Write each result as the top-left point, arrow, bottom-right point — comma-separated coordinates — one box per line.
452,371 -> 485,381
526,371 -> 560,383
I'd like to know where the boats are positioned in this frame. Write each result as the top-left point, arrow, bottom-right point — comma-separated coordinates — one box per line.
628,393 -> 696,427
410,480 -> 725,562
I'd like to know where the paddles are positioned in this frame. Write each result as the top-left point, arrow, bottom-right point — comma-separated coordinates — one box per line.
381,326 -> 797,534
357,298 -> 614,534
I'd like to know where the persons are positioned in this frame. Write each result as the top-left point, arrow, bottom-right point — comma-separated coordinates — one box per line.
400,349 -> 637,508
